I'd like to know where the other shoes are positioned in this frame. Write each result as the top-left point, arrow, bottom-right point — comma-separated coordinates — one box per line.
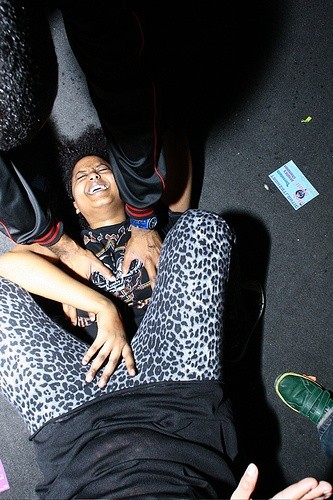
223,277 -> 264,364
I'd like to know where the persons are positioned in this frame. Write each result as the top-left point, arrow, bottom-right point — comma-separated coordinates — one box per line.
274,372 -> 333,461
0,0 -> 168,325
0,154 -> 193,388
0,209 -> 332,500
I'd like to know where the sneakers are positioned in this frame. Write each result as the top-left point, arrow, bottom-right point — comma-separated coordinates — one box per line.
274,372 -> 333,426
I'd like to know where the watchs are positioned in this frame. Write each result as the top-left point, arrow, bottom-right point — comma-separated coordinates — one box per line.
130,215 -> 157,229
